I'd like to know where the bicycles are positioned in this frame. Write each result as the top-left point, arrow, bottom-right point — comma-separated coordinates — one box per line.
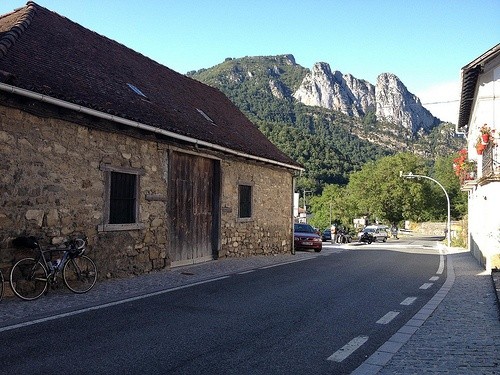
9,229 -> 98,301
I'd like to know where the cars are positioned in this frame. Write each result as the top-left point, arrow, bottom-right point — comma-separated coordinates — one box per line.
321,228 -> 331,242
294,222 -> 322,252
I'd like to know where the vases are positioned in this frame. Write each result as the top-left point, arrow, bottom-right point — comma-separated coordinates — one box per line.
478,145 -> 486,155
483,133 -> 489,143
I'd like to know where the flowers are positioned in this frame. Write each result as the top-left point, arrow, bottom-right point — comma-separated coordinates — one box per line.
476,138 -> 483,150
480,124 -> 492,134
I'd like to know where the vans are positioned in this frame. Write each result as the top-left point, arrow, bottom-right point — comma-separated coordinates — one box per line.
360,224 -> 388,242
